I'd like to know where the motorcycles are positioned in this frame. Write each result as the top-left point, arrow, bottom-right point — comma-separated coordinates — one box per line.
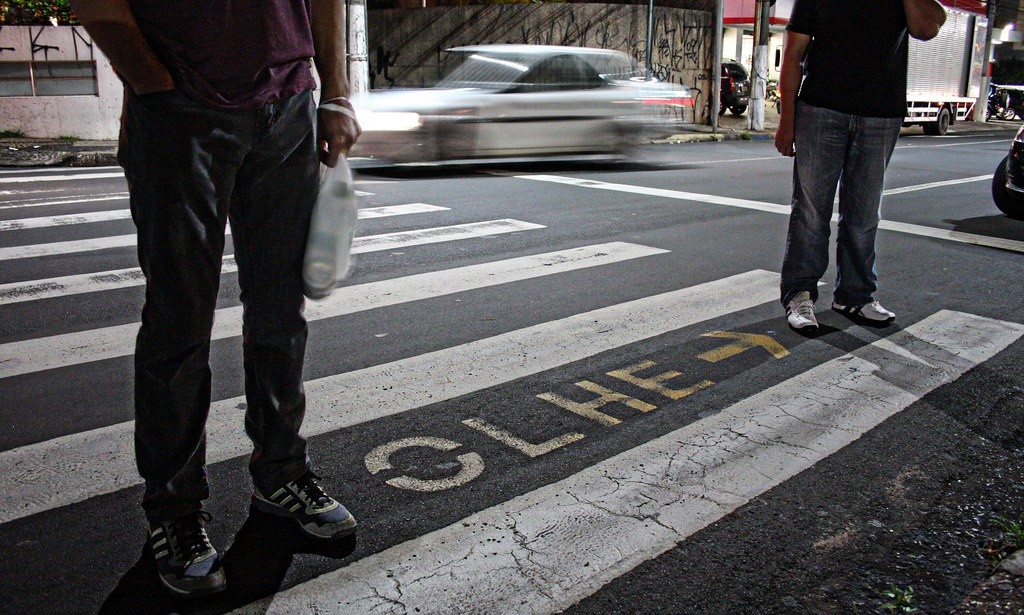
987,84 -> 1016,121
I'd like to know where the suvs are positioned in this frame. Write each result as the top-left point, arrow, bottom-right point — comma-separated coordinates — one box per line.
720,59 -> 752,120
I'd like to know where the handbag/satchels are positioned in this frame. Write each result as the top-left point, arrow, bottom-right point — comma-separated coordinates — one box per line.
301,97 -> 357,299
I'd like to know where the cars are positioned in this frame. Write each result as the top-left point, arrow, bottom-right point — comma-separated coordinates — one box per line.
992,125 -> 1024,216
338,43 -> 695,165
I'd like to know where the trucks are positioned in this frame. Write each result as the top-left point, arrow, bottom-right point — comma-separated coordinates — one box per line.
774,2 -> 987,135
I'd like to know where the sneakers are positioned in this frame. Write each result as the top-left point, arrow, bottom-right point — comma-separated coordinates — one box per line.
786,290 -> 819,329
832,298 -> 896,322
252,467 -> 357,543
148,505 -> 226,594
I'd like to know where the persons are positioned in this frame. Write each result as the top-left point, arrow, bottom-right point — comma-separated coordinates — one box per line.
774,0 -> 947,330
60,0 -> 361,598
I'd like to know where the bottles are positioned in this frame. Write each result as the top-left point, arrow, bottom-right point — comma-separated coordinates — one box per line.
301,181 -> 356,300
